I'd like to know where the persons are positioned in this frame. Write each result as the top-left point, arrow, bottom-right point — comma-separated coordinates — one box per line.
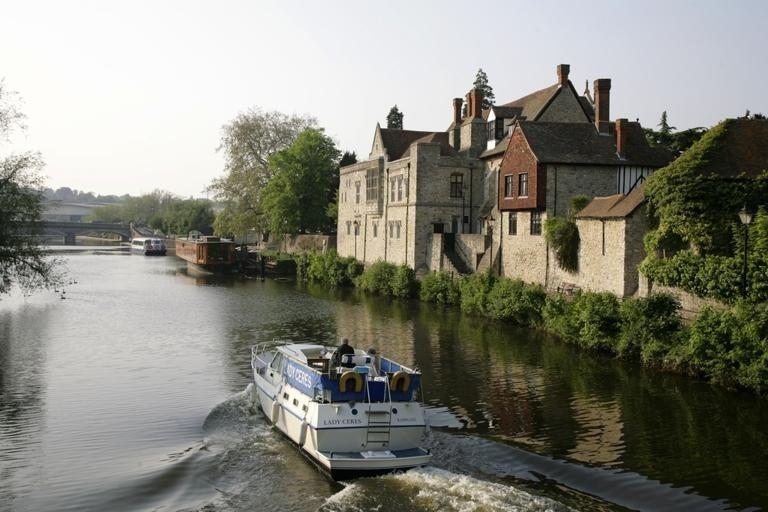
331,338 -> 356,368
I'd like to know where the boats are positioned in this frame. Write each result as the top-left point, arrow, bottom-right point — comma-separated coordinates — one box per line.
130,237 -> 167,255
173,233 -> 236,268
247,335 -> 441,481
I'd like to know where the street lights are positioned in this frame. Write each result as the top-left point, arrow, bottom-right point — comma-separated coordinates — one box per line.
736,202 -> 754,304
352,219 -> 359,261
488,212 -> 495,270
